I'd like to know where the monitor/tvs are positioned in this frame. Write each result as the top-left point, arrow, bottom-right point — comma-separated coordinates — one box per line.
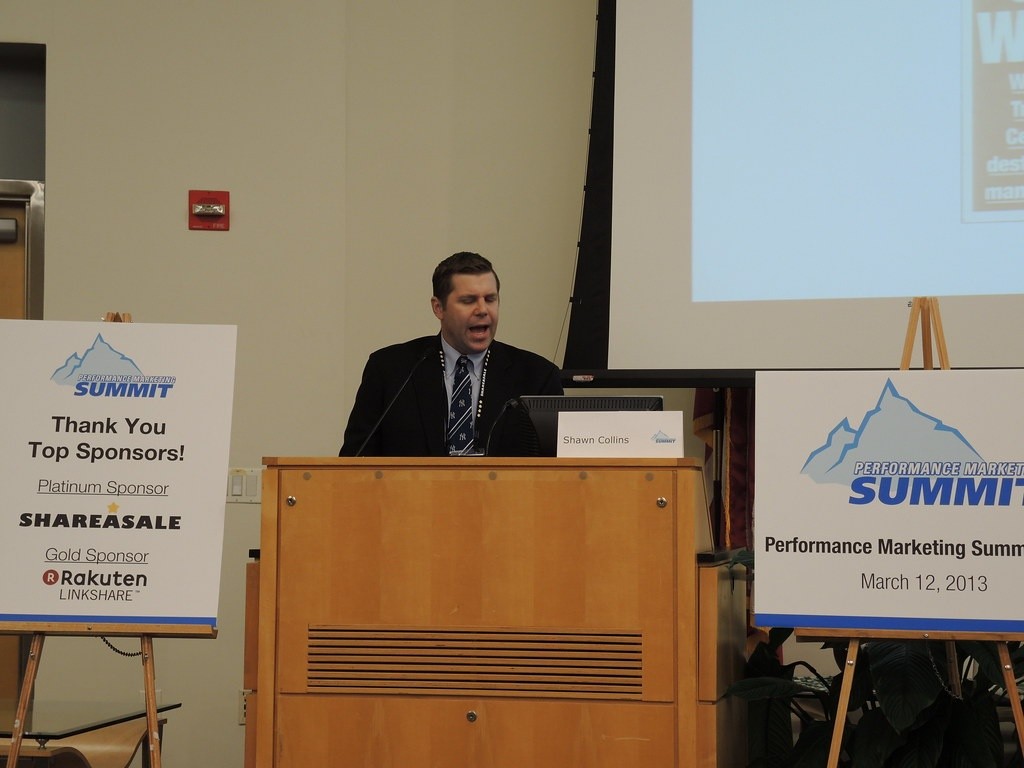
517,394 -> 665,457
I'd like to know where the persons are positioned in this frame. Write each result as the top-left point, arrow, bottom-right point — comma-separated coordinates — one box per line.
338,251 -> 560,457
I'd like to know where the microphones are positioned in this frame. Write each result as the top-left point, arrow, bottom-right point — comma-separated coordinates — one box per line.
485,399 -> 518,456
355,357 -> 428,457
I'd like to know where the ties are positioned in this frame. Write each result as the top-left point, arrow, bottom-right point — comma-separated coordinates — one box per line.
447,355 -> 474,457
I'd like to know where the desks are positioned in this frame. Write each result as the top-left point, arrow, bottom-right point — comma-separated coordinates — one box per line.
0,696 -> 182,768
